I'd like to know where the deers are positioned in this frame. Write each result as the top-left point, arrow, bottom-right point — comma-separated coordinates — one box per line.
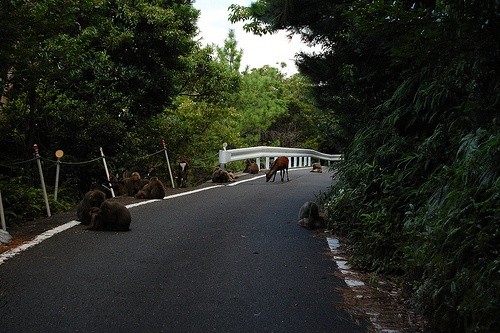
264,155 -> 290,182
242,161 -> 259,174
175,160 -> 190,188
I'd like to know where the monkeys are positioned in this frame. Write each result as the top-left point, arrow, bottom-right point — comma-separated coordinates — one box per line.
75,190 -> 132,232
211,166 -> 234,184
310,162 -> 323,173
296,201 -> 325,230
118,171 -> 166,200
